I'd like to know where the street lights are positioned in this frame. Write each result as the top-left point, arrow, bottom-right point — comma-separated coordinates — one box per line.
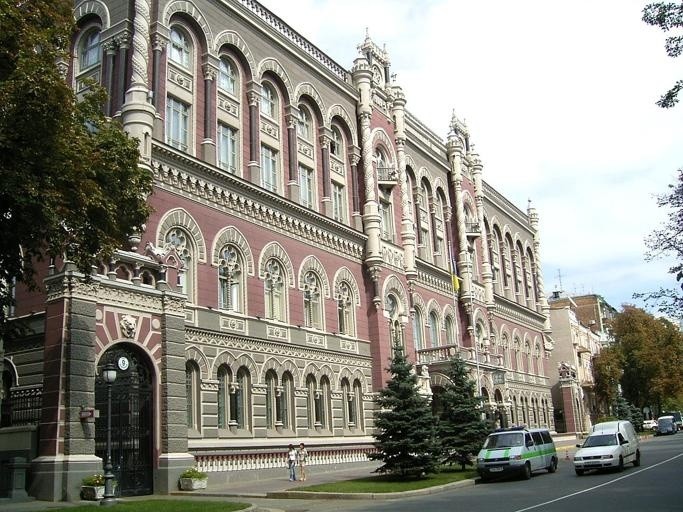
100,362 -> 119,508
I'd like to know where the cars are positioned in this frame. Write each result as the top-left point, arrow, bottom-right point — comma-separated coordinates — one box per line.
639,415 -> 682,435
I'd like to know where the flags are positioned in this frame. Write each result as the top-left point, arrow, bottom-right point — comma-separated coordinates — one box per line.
447,245 -> 460,294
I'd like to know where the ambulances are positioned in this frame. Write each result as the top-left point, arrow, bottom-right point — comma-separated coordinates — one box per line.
475,424 -> 558,481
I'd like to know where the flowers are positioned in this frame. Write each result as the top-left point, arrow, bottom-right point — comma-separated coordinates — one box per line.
180,467 -> 208,479
81,473 -> 118,487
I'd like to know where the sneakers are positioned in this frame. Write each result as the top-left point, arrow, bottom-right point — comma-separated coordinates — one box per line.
290,478 -> 306,482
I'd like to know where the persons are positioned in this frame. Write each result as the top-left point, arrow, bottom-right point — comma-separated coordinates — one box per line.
287,444 -> 298,482
296,443 -> 308,481
551,284 -> 562,298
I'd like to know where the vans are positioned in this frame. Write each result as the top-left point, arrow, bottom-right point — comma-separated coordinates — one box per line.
573,420 -> 641,475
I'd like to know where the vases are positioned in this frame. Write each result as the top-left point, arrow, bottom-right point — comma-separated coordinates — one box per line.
180,478 -> 207,490
82,486 -> 115,500
